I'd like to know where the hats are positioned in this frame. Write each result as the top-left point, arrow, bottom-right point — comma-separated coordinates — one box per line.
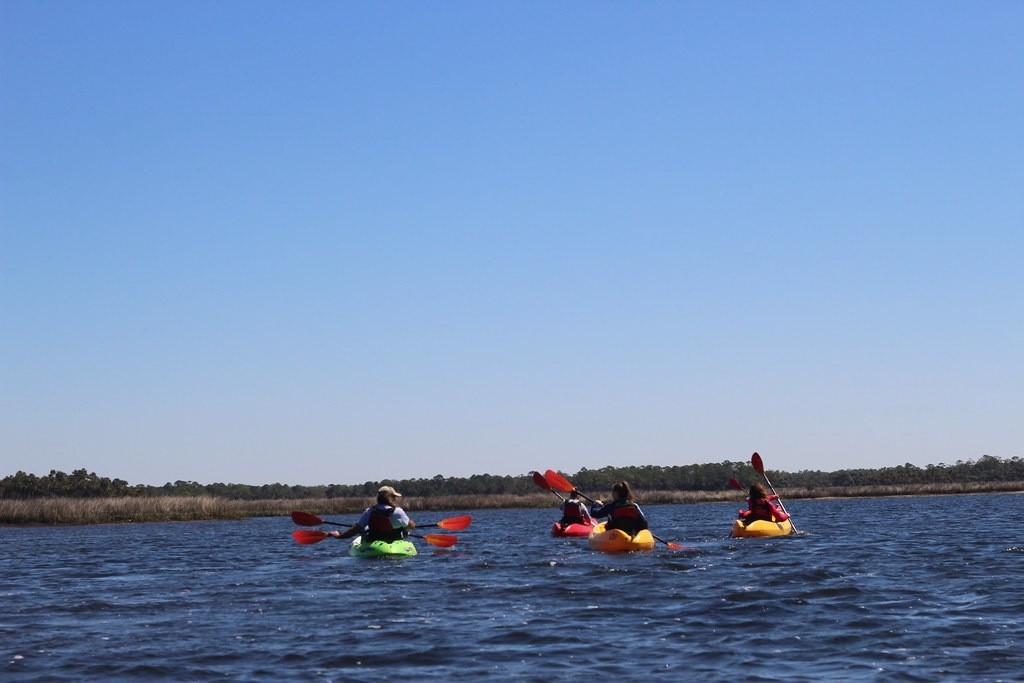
378,485 -> 402,497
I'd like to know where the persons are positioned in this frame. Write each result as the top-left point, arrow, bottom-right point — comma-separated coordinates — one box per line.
331,486 -> 415,543
739,482 -> 790,522
590,482 -> 648,538
559,487 -> 591,527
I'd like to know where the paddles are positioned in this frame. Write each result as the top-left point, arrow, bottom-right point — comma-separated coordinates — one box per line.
751,452 -> 799,535
291,511 -> 456,547
546,469 -> 677,548
532,472 -> 595,526
293,515 -> 473,545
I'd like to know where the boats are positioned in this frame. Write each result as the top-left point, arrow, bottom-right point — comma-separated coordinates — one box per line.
728,503 -> 793,538
346,535 -> 417,559
552,515 -> 600,537
588,520 -> 655,551
